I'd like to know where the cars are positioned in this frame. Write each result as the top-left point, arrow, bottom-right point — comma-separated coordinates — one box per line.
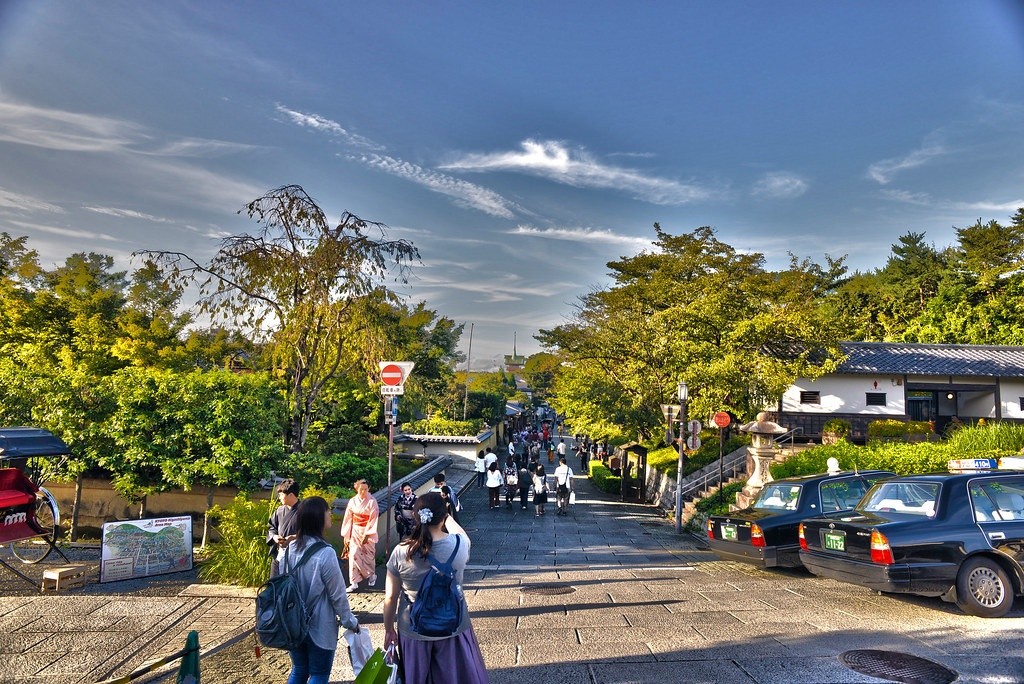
707,468 -> 901,573
801,455 -> 1024,618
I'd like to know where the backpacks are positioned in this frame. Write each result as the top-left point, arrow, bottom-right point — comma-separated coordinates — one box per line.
402,535 -> 463,637
254,541 -> 331,650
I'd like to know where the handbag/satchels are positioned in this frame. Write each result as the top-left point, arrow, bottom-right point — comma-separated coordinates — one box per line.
551,444 -> 557,451
343,627 -> 375,676
558,484 -> 568,498
354,644 -> 401,684
569,490 -> 575,505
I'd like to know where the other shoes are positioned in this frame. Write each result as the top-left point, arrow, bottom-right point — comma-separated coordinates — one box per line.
563,512 -> 567,516
346,584 -> 359,593
486,498 -> 528,513
557,509 -> 562,515
540,511 -> 546,514
368,575 -> 377,586
534,512 -> 539,516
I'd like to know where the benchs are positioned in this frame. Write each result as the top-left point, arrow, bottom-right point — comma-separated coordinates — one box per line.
0,467 -> 39,508
874,498 -> 935,515
41,562 -> 88,595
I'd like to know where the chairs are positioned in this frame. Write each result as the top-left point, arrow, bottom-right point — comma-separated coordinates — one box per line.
975,505 -> 991,521
991,492 -> 1024,521
763,497 -> 786,506
822,487 -> 837,507
844,485 -> 865,507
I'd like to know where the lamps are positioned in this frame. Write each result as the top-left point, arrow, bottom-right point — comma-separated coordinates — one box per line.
946,391 -> 955,400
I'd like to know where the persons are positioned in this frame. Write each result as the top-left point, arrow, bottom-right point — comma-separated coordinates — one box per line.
557,424 -> 563,438
341,479 -> 379,593
557,439 -> 567,460
486,462 -> 503,509
439,486 -> 459,526
475,448 -> 499,488
279,496 -> 361,684
384,491 -> 489,684
266,479 -> 301,575
503,420 -> 556,517
393,482 -> 418,541
570,433 -> 608,472
554,458 -> 574,515
427,474 -> 457,508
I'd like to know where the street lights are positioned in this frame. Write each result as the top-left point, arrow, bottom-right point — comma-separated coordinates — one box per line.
675,379 -> 688,534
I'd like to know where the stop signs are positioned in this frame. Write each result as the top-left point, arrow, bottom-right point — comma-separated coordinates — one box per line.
380,364 -> 403,386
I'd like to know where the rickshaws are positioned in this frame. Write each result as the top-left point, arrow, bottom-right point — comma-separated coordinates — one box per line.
0,426 -> 76,587
